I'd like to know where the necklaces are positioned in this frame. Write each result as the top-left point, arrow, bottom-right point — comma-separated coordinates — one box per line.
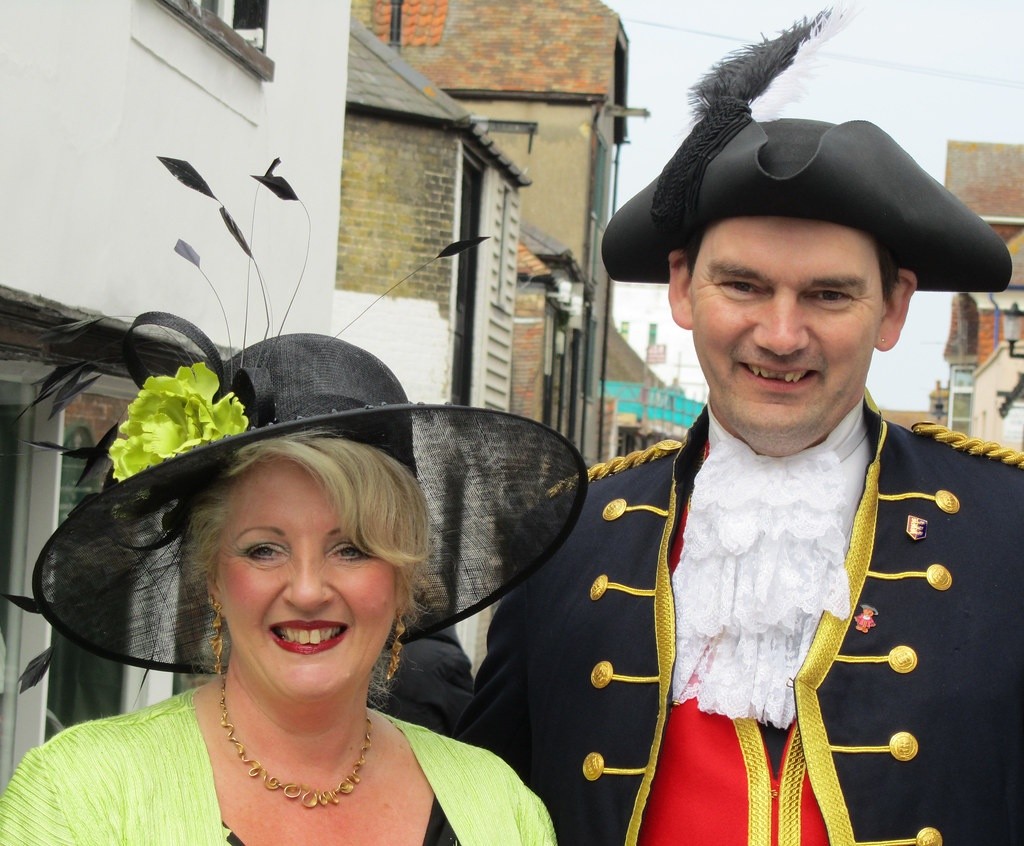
221,675 -> 372,807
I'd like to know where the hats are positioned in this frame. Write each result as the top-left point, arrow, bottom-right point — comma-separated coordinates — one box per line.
602,0 -> 1012,291
0,152 -> 590,695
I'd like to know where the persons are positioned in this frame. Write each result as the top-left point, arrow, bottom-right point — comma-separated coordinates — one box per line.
388,625 -> 471,735
0,333 -> 558,845
451,119 -> 1023,846
